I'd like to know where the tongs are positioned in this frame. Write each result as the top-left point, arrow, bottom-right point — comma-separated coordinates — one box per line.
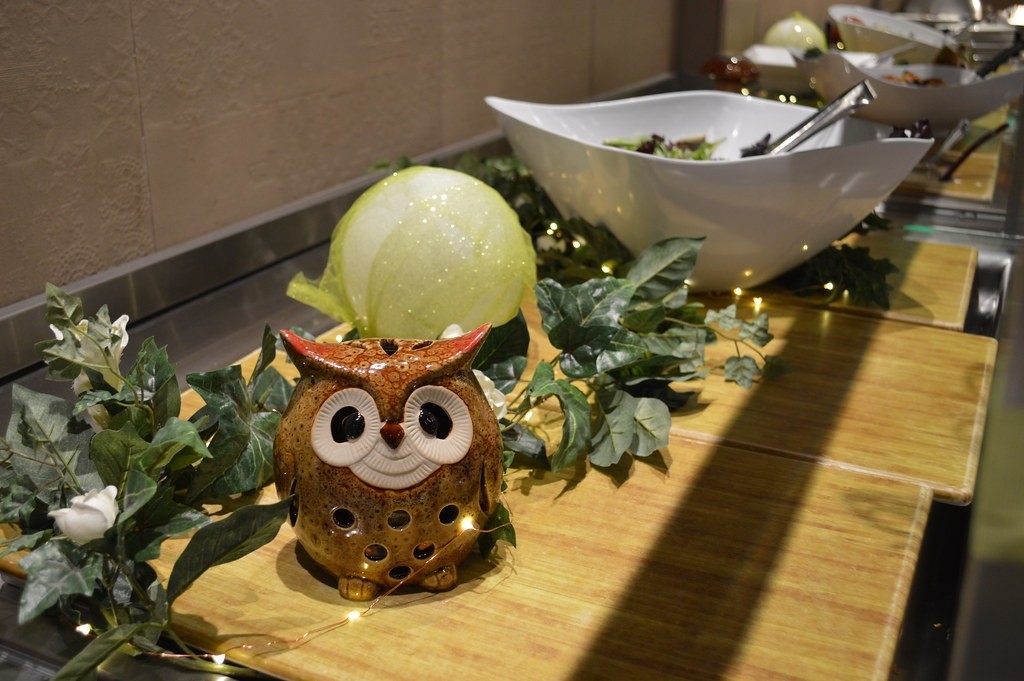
762,79 -> 878,155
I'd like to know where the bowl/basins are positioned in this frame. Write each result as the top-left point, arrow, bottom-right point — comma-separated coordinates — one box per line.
826,3 -> 959,64
743,44 -> 898,97
787,47 -> 1024,129
484,88 -> 935,294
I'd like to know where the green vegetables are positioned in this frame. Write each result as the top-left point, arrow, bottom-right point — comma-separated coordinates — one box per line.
601,132 -> 725,163
